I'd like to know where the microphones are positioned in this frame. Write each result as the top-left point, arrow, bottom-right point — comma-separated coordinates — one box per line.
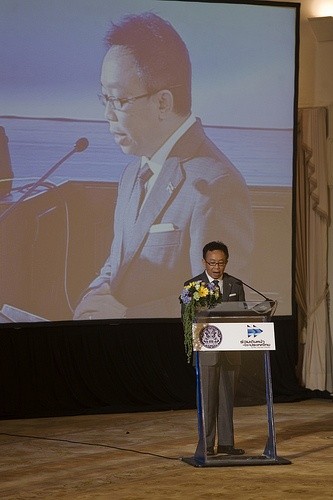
0,137 -> 88,220
223,272 -> 275,306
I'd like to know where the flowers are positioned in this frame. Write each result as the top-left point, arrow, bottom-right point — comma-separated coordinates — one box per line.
181,280 -> 222,363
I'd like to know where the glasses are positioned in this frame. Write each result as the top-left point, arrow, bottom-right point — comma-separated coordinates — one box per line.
97,84 -> 183,111
203,257 -> 228,266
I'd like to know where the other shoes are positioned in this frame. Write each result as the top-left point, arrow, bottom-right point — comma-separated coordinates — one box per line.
217,446 -> 244,455
206,449 -> 215,455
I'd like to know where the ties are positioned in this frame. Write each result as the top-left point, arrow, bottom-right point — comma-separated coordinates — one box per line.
122,163 -> 153,261
212,280 -> 219,287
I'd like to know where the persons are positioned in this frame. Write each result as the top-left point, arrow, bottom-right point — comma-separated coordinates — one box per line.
69,10 -> 252,319
178,241 -> 249,456
